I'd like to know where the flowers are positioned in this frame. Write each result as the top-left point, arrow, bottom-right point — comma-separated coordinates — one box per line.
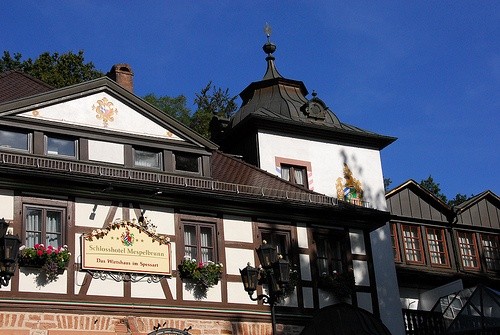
321,266 -> 354,291
84,217 -> 171,245
18,242 -> 71,282
259,261 -> 300,287
178,255 -> 222,295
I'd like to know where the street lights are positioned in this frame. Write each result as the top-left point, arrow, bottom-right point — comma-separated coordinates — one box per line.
240,240 -> 292,335
